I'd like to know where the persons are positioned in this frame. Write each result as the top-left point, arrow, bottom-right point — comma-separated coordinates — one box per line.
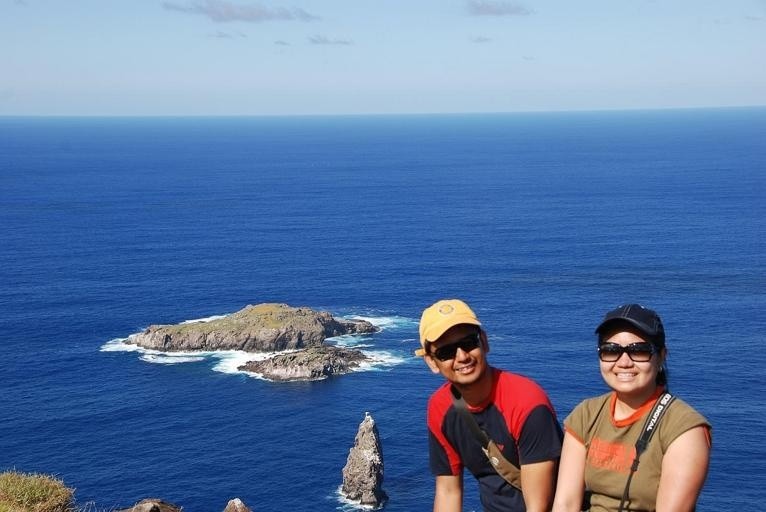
414,299 -> 561,512
552,303 -> 711,512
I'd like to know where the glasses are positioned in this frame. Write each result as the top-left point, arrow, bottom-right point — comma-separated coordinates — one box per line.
428,331 -> 480,362
597,342 -> 657,362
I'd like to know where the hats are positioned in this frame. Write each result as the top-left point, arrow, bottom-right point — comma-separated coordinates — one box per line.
415,299 -> 481,357
595,303 -> 664,334
